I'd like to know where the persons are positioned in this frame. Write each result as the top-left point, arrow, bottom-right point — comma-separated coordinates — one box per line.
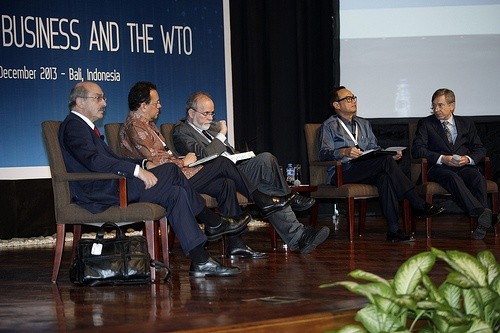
117,82 -> 299,261
316,86 -> 450,241
57,81 -> 251,277
411,89 -> 500,240
173,92 -> 330,255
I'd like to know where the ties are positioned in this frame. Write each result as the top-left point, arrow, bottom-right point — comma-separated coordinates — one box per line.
441,121 -> 453,146
201,130 -> 234,155
92,126 -> 101,138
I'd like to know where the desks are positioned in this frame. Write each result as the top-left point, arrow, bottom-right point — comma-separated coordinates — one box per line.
288,184 -> 319,192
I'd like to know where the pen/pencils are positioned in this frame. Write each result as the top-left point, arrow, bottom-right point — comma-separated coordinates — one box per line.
178,154 -> 200,158
210,121 -> 218,124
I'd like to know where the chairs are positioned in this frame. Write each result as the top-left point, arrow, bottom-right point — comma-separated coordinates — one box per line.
304,123 -> 412,244
408,124 -> 500,238
41,120 -> 275,284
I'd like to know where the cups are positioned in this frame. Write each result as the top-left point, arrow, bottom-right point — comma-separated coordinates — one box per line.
293,164 -> 302,180
279,166 -> 283,175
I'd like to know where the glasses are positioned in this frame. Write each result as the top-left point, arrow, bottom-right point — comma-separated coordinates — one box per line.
336,95 -> 358,103
190,106 -> 216,118
77,96 -> 107,103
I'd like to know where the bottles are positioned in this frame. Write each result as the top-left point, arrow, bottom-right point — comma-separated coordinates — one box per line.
286,163 -> 294,181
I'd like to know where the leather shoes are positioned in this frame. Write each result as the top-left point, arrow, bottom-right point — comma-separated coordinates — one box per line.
386,228 -> 417,243
188,255 -> 240,277
417,200 -> 445,221
256,192 -> 297,218
471,208 -> 500,240
204,213 -> 251,242
298,226 -> 330,253
225,241 -> 269,258
291,192 -> 315,211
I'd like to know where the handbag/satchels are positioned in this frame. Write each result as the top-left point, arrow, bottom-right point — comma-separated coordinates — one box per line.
70,221 -> 151,286
